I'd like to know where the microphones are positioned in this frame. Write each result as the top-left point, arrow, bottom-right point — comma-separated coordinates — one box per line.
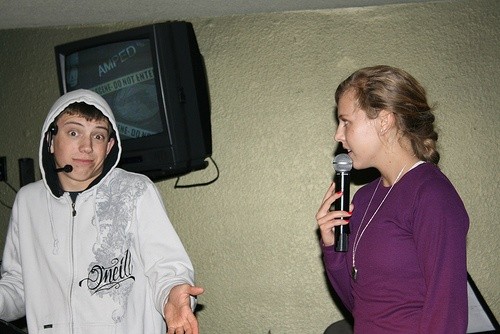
332,154 -> 353,252
56,165 -> 73,173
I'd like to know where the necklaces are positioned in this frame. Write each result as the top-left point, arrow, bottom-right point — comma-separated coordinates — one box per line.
352,154 -> 417,279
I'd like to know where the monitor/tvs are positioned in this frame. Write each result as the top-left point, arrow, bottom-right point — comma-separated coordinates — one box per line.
54,21 -> 212,176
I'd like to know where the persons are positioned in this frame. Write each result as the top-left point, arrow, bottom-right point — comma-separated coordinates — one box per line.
315,65 -> 470,334
0,89 -> 204,334
